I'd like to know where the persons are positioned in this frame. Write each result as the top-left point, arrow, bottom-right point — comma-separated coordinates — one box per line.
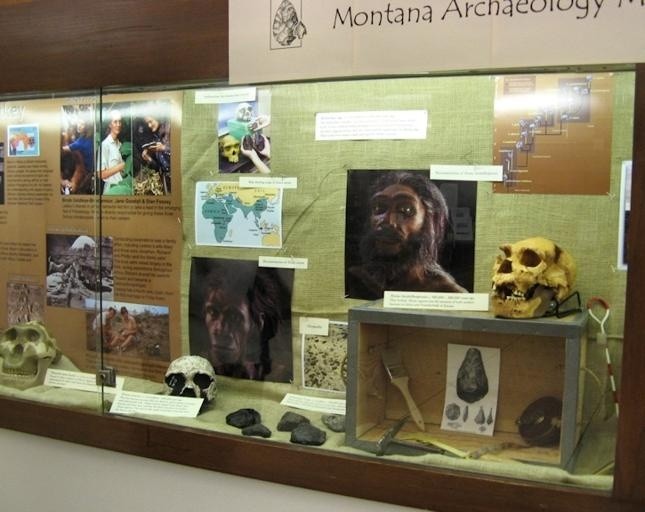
93,307 -> 116,343
345,171 -> 470,300
198,259 -> 289,381
109,307 -> 138,352
241,131 -> 270,173
60,115 -> 170,196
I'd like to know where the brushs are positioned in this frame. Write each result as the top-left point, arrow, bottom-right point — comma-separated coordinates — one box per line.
382,349 -> 424,431
